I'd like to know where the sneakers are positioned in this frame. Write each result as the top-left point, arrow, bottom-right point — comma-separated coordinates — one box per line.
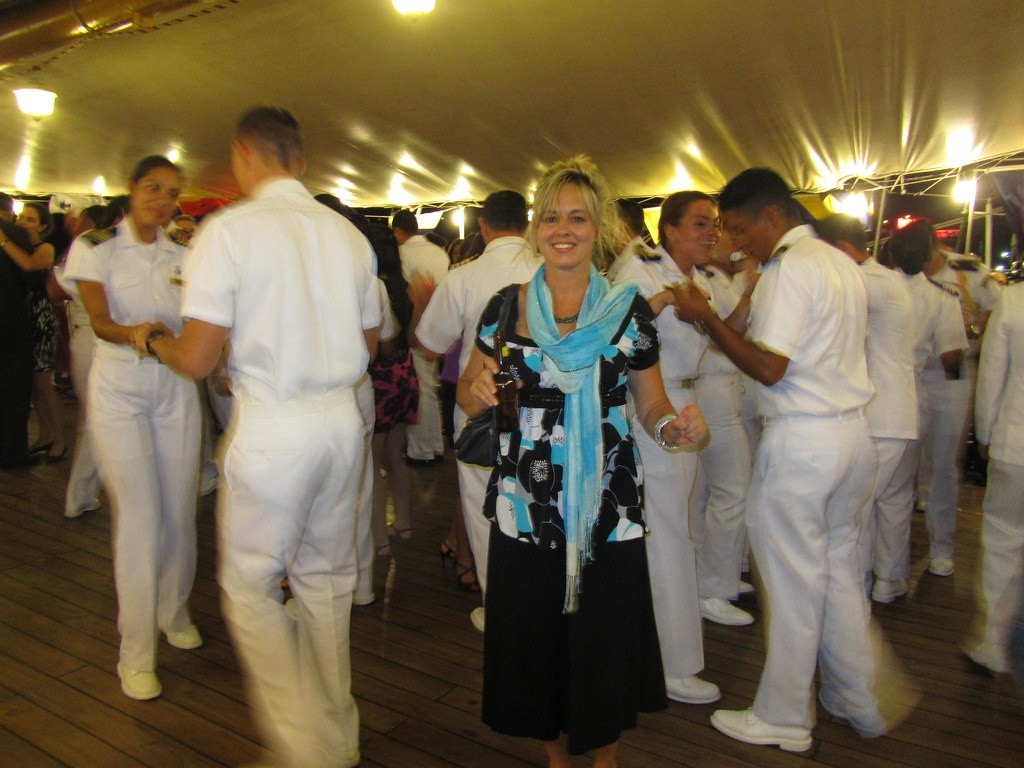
118,662 -> 161,700
165,624 -> 202,650
666,676 -> 721,704
699,598 -> 754,625
929,558 -> 954,576
818,691 -> 886,738
710,706 -> 812,752
872,577 -> 908,603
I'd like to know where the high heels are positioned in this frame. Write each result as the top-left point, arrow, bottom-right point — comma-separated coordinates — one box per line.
456,561 -> 479,591
440,541 -> 459,569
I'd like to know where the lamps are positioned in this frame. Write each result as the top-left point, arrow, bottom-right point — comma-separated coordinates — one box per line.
12,76 -> 58,122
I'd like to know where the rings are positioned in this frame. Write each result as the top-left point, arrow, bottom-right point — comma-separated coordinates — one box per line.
484,360 -> 485,369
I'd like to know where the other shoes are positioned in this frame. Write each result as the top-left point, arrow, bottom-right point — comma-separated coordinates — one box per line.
739,580 -> 755,593
28,439 -> 53,452
65,500 -> 101,518
41,444 -> 67,462
471,607 -> 485,632
406,455 -> 443,466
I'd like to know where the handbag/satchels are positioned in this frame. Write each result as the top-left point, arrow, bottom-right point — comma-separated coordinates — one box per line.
450,283 -> 520,466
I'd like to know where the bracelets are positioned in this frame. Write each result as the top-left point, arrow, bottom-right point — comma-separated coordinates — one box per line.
654,415 -> 681,449
0,238 -> 10,248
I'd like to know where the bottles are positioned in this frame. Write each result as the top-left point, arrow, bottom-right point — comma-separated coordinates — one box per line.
492,329 -> 521,432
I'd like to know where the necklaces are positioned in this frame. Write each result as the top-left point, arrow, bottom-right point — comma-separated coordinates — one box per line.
553,313 -> 577,323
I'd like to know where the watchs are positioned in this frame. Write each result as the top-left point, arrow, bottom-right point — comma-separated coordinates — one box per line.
970,324 -> 979,337
146,330 -> 165,356
693,320 -> 704,332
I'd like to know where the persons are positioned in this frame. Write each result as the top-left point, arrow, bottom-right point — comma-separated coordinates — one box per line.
0,202 -> 68,464
61,155 -> 203,700
137,106 -> 382,768
0,191 -> 43,467
607,168 -> 1024,751
454,161 -> 710,768
64,196 -> 232,519
315,190 -> 545,631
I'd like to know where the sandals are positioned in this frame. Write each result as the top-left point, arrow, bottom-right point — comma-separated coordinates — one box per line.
375,543 -> 391,560
387,523 -> 413,543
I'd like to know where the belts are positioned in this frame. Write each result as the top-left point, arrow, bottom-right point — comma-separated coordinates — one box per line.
663,378 -> 695,390
